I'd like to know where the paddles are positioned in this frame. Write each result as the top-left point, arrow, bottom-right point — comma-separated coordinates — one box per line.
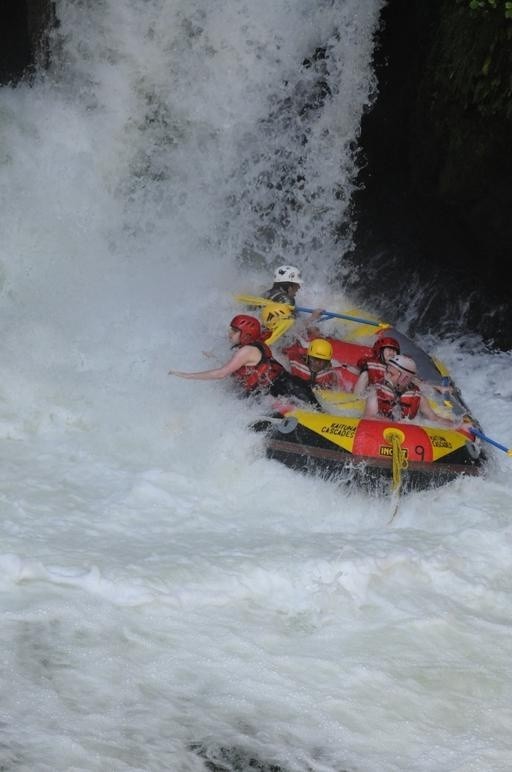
264,315 -> 335,346
313,390 -> 366,416
236,295 -> 389,329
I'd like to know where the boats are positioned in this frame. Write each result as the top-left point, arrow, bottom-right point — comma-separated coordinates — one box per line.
242,310 -> 485,497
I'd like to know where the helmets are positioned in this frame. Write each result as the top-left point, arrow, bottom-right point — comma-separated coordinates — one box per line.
306,339 -> 333,364
373,337 -> 416,393
273,265 -> 302,283
231,316 -> 260,345
